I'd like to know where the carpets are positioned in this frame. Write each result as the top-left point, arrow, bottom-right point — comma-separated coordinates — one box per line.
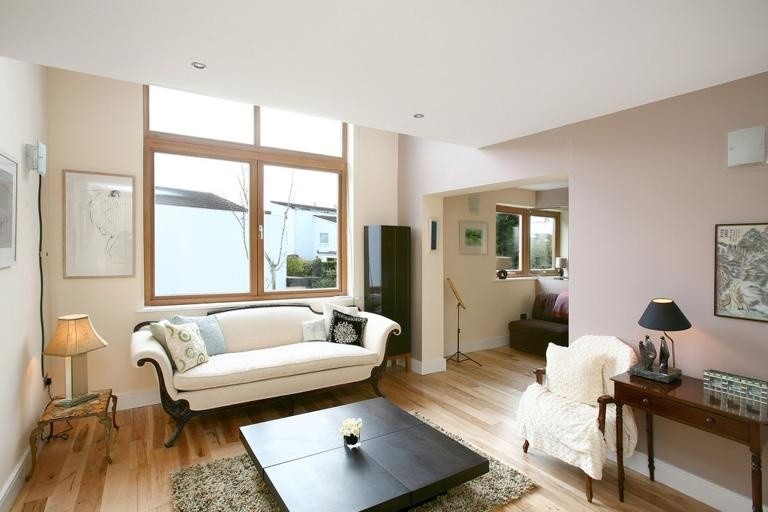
171,411 -> 535,512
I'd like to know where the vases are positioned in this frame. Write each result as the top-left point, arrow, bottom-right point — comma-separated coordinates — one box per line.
345,435 -> 358,447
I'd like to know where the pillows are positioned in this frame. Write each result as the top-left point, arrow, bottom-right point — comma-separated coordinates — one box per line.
543,340 -> 609,408
301,302 -> 369,349
145,314 -> 227,374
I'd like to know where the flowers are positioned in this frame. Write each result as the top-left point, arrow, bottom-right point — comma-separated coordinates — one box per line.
341,417 -> 365,441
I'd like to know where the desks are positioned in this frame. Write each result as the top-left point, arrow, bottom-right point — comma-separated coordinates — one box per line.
610,366 -> 767,512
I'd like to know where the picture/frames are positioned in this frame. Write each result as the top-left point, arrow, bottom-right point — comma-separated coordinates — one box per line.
1,153 -> 19,272
459,220 -> 489,255
712,222 -> 768,322
62,169 -> 137,279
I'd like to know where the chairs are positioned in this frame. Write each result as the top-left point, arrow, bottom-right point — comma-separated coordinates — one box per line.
520,334 -> 644,503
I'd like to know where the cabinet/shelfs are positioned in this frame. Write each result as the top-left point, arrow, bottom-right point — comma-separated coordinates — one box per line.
365,224 -> 413,375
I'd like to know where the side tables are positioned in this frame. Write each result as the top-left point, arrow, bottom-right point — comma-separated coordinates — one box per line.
23,388 -> 121,484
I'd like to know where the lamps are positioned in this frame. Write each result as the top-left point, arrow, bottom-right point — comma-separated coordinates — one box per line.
556,256 -> 567,280
44,313 -> 109,407
637,296 -> 692,369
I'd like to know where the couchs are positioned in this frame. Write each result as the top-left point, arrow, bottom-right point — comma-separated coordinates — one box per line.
508,292 -> 568,357
128,299 -> 402,449
